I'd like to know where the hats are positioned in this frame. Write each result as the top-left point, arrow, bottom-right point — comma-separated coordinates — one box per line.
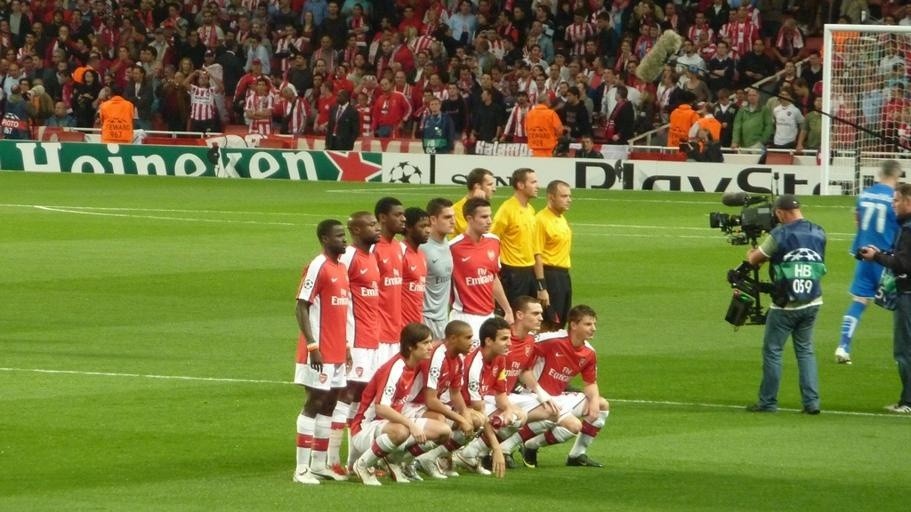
773,193 -> 800,208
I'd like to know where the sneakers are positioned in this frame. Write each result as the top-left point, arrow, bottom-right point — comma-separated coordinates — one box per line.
346,452 -> 460,486
294,465 -> 348,485
565,454 -> 603,467
518,440 -> 538,468
454,444 -> 519,474
745,404 -> 762,412
835,348 -> 851,364
885,404 -> 910,412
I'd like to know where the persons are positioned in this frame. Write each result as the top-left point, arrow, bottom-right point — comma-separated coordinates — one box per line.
293,167 -> 611,485
833,158 -> 903,365
0,2 -> 911,164
859,182 -> 910,414
746,194 -> 829,416
